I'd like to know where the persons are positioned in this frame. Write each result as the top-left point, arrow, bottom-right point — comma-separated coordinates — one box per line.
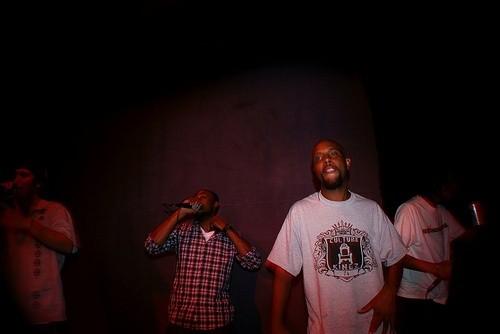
144,188 -> 263,334
0,164 -> 80,334
394,155 -> 481,334
267,138 -> 410,334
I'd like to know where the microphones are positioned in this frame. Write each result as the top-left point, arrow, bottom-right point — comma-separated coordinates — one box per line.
426,277 -> 442,293
169,202 -> 199,210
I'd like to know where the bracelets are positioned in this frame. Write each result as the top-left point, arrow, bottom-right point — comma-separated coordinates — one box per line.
434,262 -> 440,277
27,218 -> 35,235
222,223 -> 233,233
